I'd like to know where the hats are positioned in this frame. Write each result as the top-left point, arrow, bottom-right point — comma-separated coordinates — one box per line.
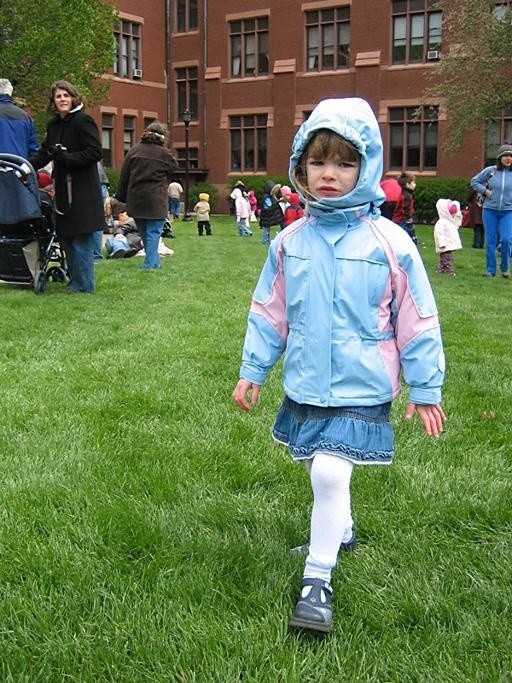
449,204 -> 457,214
496,144 -> 512,159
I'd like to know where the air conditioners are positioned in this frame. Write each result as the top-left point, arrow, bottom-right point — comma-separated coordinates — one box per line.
132,69 -> 142,77
428,51 -> 441,59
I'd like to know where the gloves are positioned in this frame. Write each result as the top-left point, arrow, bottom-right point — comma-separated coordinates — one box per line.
46,144 -> 60,160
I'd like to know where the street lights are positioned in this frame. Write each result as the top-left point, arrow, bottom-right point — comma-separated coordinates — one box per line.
180,106 -> 195,221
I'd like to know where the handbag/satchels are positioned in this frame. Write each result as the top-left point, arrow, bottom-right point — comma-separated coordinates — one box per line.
475,165 -> 497,208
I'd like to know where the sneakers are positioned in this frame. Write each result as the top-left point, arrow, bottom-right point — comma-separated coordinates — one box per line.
502,271 -> 510,277
124,248 -> 138,258
483,272 -> 496,276
108,248 -> 125,259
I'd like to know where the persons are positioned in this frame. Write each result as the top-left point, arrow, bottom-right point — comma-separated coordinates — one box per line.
37,173 -> 55,228
194,193 -> 212,236
167,178 -> 183,219
92,162 -> 109,262
231,97 -> 446,636
243,195 -> 251,228
105,215 -> 175,259
470,145 -> 512,278
392,173 -> 417,245
433,198 -> 463,277
230,180 -> 252,237
247,191 -> 258,222
116,120 -> 179,271
28,80 -> 104,293
286,193 -> 304,226
259,180 -> 284,246
465,180 -> 489,249
0,78 -> 40,185
278,185 -> 292,230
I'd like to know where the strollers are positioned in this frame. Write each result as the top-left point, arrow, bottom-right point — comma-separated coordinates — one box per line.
0,153 -> 72,295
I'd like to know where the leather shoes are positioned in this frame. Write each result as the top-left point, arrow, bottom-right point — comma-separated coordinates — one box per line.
290,531 -> 357,556
290,577 -> 337,631
